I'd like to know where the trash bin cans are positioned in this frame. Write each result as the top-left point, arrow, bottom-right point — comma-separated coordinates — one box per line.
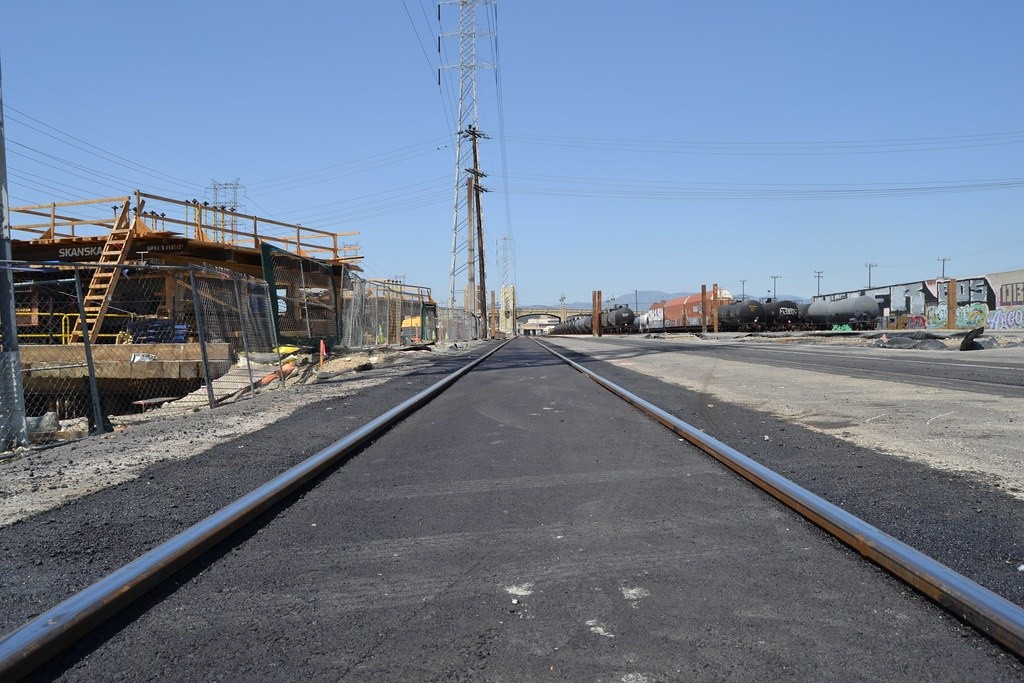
401,316 -> 432,340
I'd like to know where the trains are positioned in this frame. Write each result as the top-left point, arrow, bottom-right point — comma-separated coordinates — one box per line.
632,288 -> 880,331
544,303 -> 637,335
